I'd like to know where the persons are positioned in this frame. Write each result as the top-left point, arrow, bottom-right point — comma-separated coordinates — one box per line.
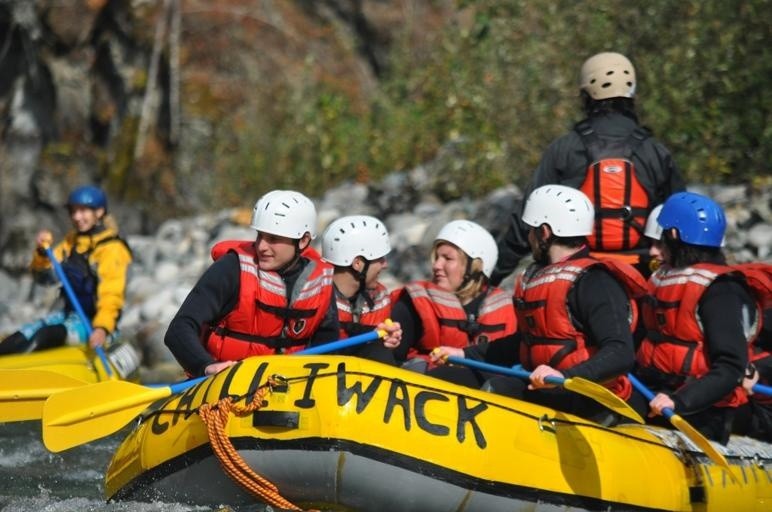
391,219 -> 517,374
429,184 -> 636,427
620,192 -> 756,444
489,51 -> 687,285
0,184 -> 132,355
164,190 -> 402,377
645,204 -> 670,269
321,214 -> 391,364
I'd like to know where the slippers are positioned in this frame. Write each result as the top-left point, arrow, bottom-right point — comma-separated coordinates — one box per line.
575,120 -> 654,247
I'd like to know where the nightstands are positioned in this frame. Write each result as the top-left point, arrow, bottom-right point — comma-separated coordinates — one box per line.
628,372 -> 743,487
0,368 -> 175,423
42,318 -> 392,453
433,347 -> 646,427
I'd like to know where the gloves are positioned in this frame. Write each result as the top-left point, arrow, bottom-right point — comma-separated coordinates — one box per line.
105,350 -> 772,512
1,340 -> 144,423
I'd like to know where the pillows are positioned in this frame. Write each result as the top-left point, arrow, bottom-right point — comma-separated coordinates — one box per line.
63,185 -> 108,212
578,52 -> 636,100
656,191 -> 727,248
321,214 -> 392,267
248,190 -> 318,240
521,184 -> 594,237
643,204 -> 725,248
432,219 -> 498,280
